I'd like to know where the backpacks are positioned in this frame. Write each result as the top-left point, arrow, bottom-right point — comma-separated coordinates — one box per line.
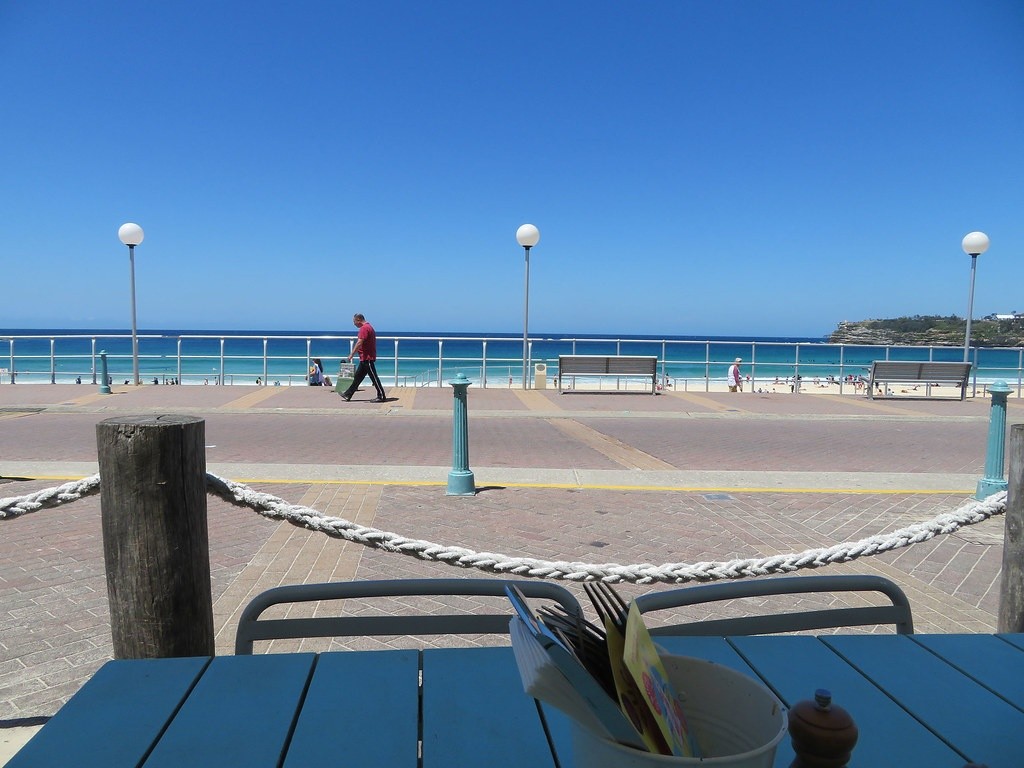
256,380 -> 258,384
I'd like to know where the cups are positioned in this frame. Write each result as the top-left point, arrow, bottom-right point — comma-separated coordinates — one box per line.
786,687 -> 858,767
567,652 -> 789,768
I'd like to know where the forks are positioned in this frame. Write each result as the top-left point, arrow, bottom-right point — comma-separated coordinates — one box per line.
536,604 -> 610,676
582,581 -> 628,638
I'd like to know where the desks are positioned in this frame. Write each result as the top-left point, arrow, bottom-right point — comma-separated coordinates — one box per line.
3,633 -> 1024,768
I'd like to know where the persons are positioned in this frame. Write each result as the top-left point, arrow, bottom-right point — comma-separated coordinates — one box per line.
139,378 -> 142,384
75,375 -> 81,384
812,375 -> 865,388
108,377 -> 112,384
124,379 -> 129,384
728,358 -> 742,392
338,313 -> 386,403
214,377 -> 218,385
878,389 -> 893,396
165,377 -> 178,385
745,373 -> 750,382
653,373 -> 671,391
204,378 -> 208,385
309,358 -> 323,386
791,375 -> 802,393
274,381 -> 280,386
256,377 -> 261,385
151,378 -> 158,384
340,360 -> 346,363
753,388 -> 776,393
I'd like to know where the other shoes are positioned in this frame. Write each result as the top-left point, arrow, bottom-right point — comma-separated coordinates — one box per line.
338,391 -> 350,401
370,397 -> 386,403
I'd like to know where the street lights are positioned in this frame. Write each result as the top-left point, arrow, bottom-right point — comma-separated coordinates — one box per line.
960,230 -> 989,403
119,222 -> 144,385
514,223 -> 541,390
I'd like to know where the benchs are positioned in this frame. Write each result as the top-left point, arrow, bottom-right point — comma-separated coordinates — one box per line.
858,360 -> 972,401
558,355 -> 658,396
